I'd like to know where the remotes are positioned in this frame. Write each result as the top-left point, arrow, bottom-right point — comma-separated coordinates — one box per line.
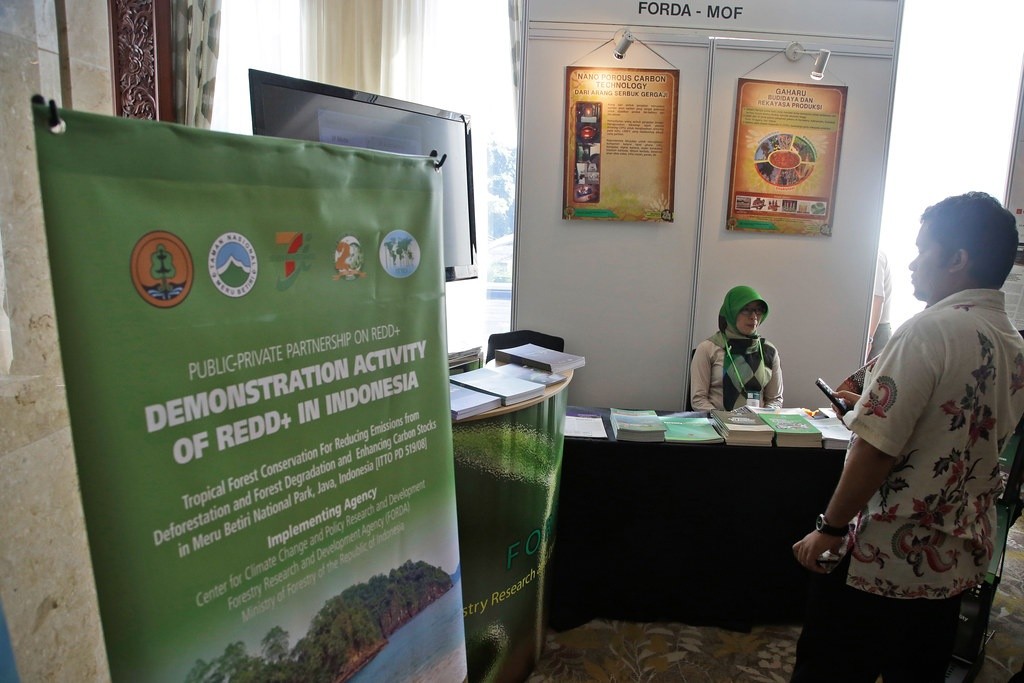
815,378 -> 850,415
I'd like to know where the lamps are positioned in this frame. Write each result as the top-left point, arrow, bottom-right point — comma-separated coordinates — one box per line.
784,42 -> 830,81
612,28 -> 634,61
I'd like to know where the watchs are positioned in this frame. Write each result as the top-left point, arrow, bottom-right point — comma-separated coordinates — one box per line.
869,336 -> 873,343
816,514 -> 849,537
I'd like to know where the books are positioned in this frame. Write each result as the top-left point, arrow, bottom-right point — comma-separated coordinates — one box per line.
564,405 -> 853,450
448,343 -> 586,421
999,264 -> 1024,331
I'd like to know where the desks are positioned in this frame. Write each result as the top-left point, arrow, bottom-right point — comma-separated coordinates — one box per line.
545,404 -> 846,634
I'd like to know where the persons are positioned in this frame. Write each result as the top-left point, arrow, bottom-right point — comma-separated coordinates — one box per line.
689,286 -> 784,413
866,248 -> 893,366
792,191 -> 1024,683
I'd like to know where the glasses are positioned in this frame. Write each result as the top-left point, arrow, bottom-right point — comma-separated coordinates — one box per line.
740,307 -> 763,315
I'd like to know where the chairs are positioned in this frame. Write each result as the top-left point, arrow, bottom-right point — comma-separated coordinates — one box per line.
686,350 -> 697,413
486,330 -> 564,363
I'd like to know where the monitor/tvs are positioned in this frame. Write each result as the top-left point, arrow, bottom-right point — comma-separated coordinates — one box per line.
247,67 -> 479,282
